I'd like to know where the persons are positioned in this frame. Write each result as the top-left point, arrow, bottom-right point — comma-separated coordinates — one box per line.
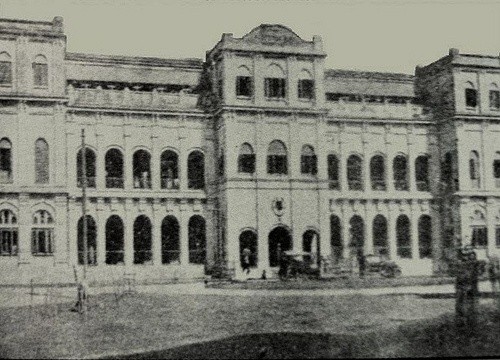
241,241 -> 312,283
